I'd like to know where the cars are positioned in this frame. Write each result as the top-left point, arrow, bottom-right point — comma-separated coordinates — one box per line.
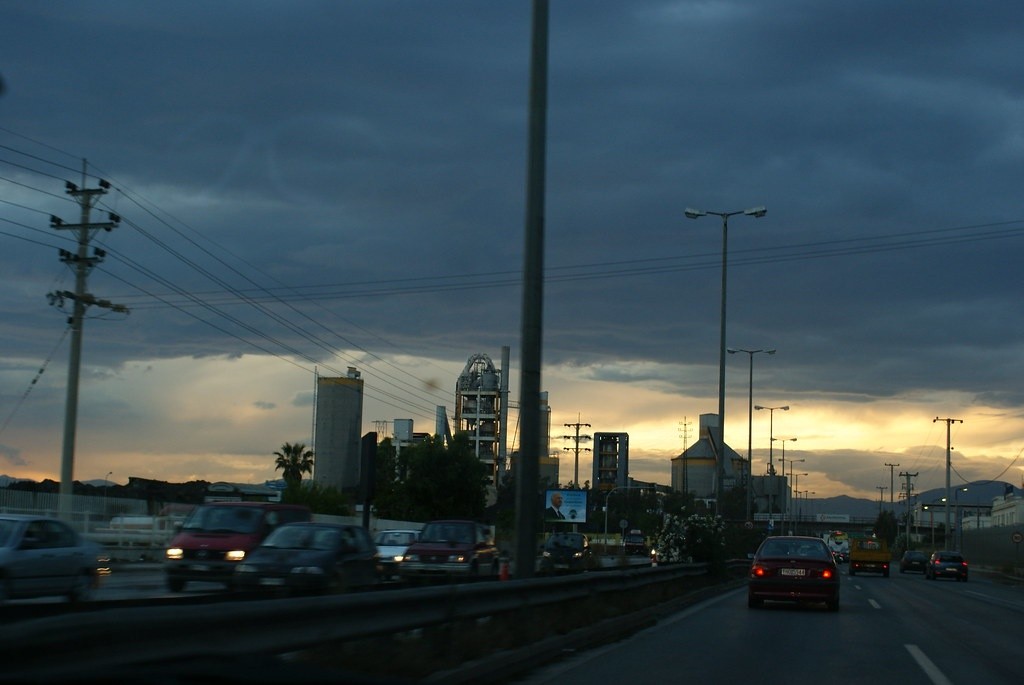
103,499 -> 678,602
826,536 -> 850,565
927,551 -> 969,583
0,513 -> 113,616
746,534 -> 842,613
899,549 -> 929,575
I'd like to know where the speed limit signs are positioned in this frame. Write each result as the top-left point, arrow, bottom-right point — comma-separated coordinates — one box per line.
1012,532 -> 1023,544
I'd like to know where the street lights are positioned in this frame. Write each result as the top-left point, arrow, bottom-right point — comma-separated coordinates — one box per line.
725,346 -> 777,562
683,203 -> 769,580
929,497 -> 948,550
770,437 -> 816,537
954,487 -> 968,551
753,404 -> 790,536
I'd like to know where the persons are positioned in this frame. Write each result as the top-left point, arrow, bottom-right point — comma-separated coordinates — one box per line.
546,492 -> 565,519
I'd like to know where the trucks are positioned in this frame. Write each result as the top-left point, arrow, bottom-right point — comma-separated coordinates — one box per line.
848,537 -> 894,578
